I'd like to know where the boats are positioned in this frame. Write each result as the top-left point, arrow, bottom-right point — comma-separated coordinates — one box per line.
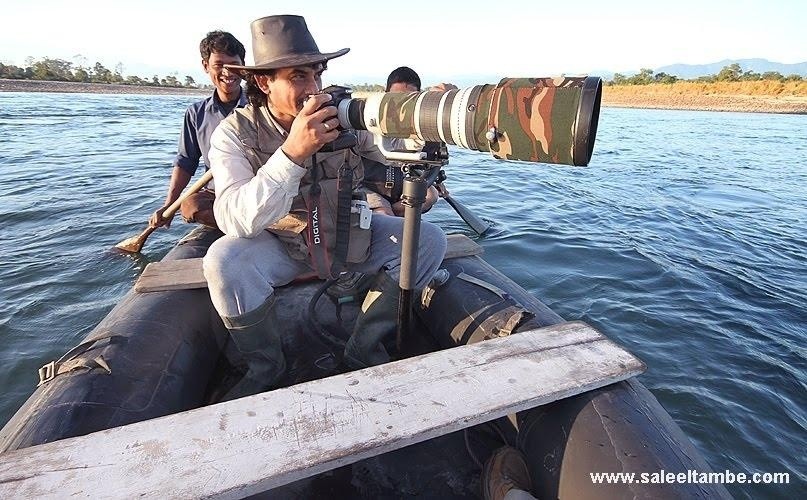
0,226 -> 733,498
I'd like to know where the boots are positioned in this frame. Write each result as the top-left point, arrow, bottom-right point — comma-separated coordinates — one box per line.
342,268 -> 401,370
216,289 -> 286,406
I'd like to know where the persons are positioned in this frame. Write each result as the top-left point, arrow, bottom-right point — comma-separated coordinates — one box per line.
147,28 -> 250,229
201,15 -> 446,404
479,445 -> 543,500
352,67 -> 449,219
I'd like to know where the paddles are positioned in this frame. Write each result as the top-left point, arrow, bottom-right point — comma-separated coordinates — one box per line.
114,167 -> 212,252
413,168 -> 490,235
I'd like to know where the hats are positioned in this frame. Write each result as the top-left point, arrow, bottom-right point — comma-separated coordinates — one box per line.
221,12 -> 350,81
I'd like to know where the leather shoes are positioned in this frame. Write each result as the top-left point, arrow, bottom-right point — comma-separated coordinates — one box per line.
480,445 -> 533,499
